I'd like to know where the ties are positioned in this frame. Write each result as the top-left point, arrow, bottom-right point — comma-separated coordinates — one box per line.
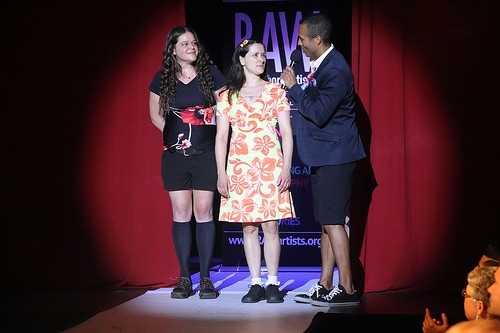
306,69 -> 317,88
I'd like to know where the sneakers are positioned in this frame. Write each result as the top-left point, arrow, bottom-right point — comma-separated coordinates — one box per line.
310,285 -> 361,306
198,277 -> 219,299
172,276 -> 193,298
266,284 -> 285,302
294,280 -> 333,303
242,284 -> 266,305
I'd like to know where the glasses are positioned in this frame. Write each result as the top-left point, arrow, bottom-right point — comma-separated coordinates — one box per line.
461,288 -> 483,302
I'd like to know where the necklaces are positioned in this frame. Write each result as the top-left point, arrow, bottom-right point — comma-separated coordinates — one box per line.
181,70 -> 193,79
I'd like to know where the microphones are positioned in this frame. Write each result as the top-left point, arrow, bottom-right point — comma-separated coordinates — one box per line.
281,50 -> 301,88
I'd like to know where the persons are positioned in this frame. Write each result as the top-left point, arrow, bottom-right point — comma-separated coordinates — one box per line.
280,14 -> 367,307
422,245 -> 500,333
149,26 -> 229,299
215,40 -> 296,303
